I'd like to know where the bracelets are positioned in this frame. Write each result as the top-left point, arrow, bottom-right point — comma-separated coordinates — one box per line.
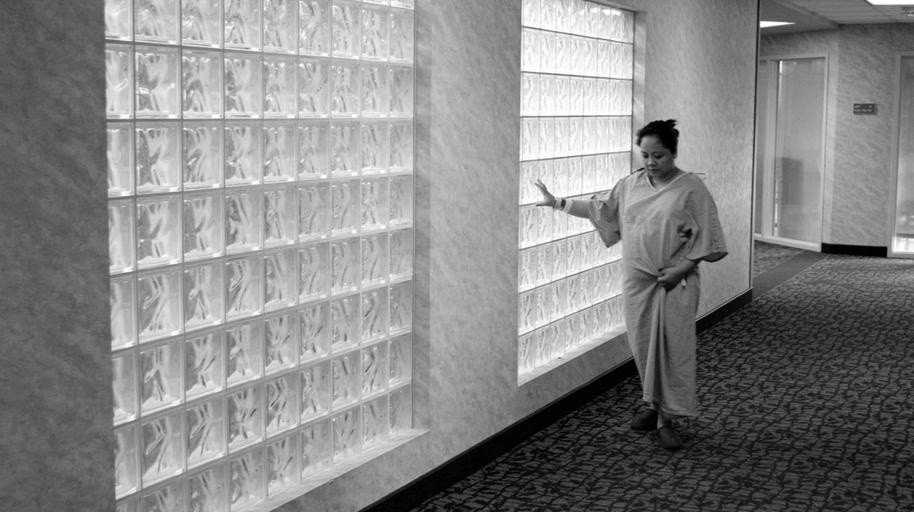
552,198 -> 573,214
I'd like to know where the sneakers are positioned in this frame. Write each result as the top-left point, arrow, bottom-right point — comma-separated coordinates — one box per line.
658,426 -> 681,450
631,408 -> 659,431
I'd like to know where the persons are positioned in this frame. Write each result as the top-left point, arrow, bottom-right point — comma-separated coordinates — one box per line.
532,118 -> 730,450
